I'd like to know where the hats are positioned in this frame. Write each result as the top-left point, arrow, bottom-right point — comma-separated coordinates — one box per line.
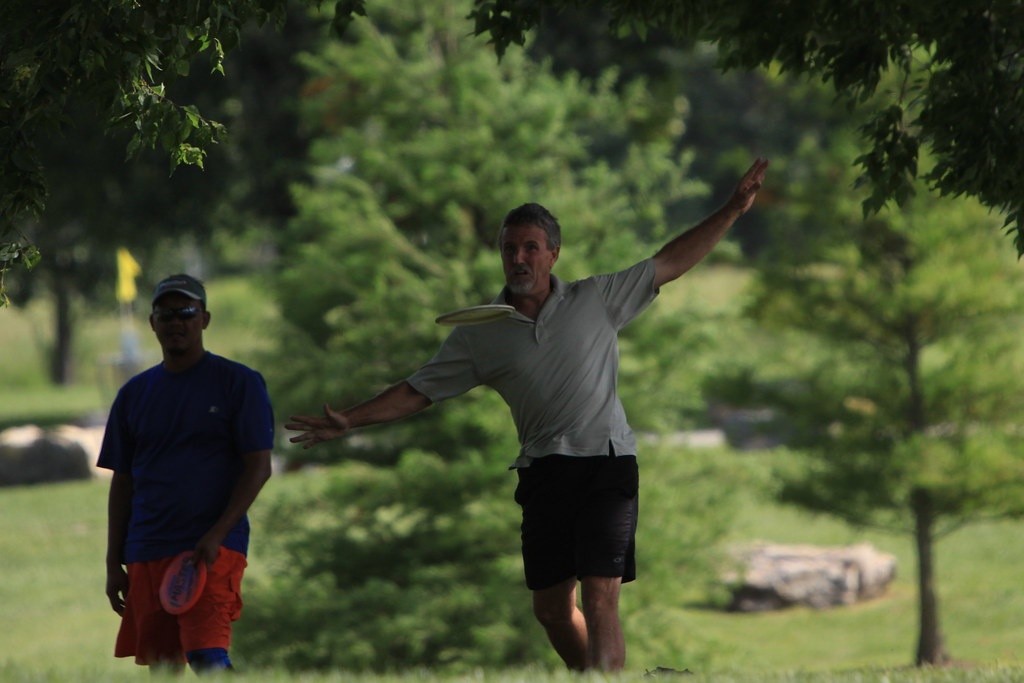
152,274 -> 206,309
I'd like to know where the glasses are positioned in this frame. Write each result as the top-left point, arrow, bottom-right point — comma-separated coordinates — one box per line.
155,306 -> 198,321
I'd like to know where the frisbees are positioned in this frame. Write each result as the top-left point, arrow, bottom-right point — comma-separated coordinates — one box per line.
159,552 -> 207,614
435,304 -> 515,326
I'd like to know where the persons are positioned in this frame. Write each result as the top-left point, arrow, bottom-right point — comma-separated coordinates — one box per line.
284,158 -> 770,675
97,274 -> 275,674
